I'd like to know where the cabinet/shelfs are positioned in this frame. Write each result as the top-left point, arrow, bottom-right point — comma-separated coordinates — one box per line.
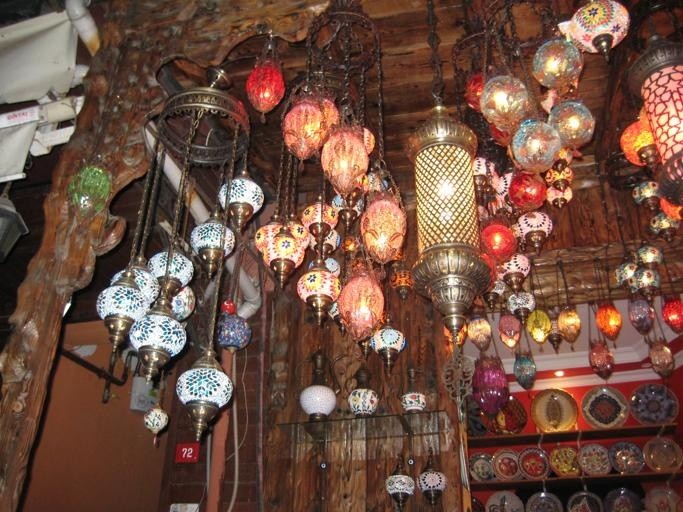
458,420 -> 682,511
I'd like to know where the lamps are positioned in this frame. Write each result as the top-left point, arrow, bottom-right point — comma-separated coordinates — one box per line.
61,1 -> 682,445
